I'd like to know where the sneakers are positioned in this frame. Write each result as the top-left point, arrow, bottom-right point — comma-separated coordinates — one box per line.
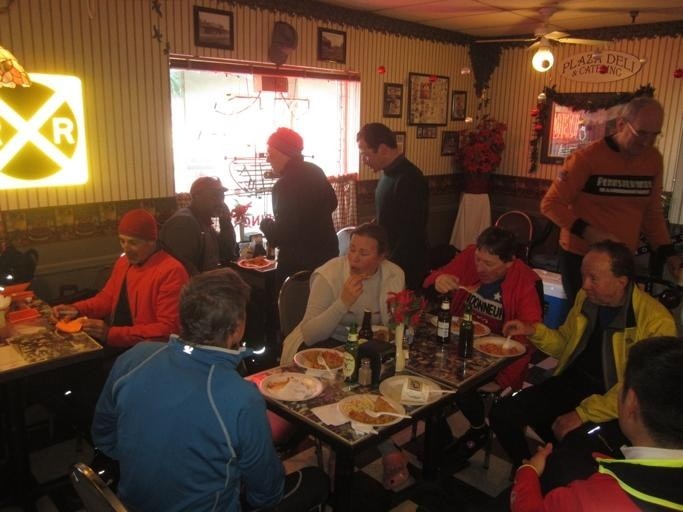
458,425 -> 490,461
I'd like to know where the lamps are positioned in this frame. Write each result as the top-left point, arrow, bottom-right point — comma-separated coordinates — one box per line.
0,38 -> 32,89
531,30 -> 554,74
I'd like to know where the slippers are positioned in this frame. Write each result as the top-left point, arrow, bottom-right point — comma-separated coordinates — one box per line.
381,451 -> 409,490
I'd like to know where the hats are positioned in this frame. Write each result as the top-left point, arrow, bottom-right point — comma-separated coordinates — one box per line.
189,175 -> 228,198
118,207 -> 158,241
266,126 -> 304,160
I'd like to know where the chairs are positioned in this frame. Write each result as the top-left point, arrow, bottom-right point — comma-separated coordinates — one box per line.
275,269 -> 316,339
67,463 -> 127,510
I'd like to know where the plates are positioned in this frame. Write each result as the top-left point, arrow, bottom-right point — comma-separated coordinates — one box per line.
339,395 -> 406,427
472,336 -> 526,358
260,372 -> 323,401
356,326 -> 388,333
378,376 -> 442,405
293,348 -> 344,367
430,314 -> 490,337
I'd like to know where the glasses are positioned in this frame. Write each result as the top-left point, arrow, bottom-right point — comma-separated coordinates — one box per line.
625,120 -> 664,143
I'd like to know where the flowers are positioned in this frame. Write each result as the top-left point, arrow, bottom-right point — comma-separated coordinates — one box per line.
449,112 -> 507,179
523,82 -> 653,175
229,198 -> 252,227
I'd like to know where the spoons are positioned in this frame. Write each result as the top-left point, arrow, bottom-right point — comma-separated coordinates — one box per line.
316,352 -> 334,379
502,334 -> 512,353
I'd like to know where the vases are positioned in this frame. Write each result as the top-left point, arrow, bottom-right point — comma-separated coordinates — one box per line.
238,224 -> 244,244
463,174 -> 490,195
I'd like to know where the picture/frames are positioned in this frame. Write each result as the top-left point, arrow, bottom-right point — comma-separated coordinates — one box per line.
191,3 -> 236,52
316,24 -> 348,66
537,90 -> 635,165
377,72 -> 469,163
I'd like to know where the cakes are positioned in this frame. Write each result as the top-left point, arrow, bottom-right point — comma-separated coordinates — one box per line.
400,378 -> 430,404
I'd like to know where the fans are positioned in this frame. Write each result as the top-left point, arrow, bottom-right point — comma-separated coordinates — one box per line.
470,5 -> 617,52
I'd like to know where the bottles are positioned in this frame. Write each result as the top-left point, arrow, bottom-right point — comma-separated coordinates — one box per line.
458,301 -> 473,357
402,337 -> 410,360
435,294 -> 451,343
343,307 -> 375,387
265,240 -> 275,259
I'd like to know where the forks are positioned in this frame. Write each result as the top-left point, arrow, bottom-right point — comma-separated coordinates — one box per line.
362,408 -> 413,420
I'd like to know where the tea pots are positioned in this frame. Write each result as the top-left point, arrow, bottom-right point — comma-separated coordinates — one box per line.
0,246 -> 39,286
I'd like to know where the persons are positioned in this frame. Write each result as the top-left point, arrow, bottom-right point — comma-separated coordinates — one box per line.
540,97 -> 682,303
491,239 -> 677,483
509,336 -> 682,512
259,127 -> 341,281
282,227 -> 411,488
161,177 -> 241,275
421,227 -> 542,466
49,209 -> 188,354
88,264 -> 331,511
355,122 -> 430,295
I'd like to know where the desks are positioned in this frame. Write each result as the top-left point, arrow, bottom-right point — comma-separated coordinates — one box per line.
240,321 -> 528,512
450,193 -> 494,261
2,282 -> 107,420
